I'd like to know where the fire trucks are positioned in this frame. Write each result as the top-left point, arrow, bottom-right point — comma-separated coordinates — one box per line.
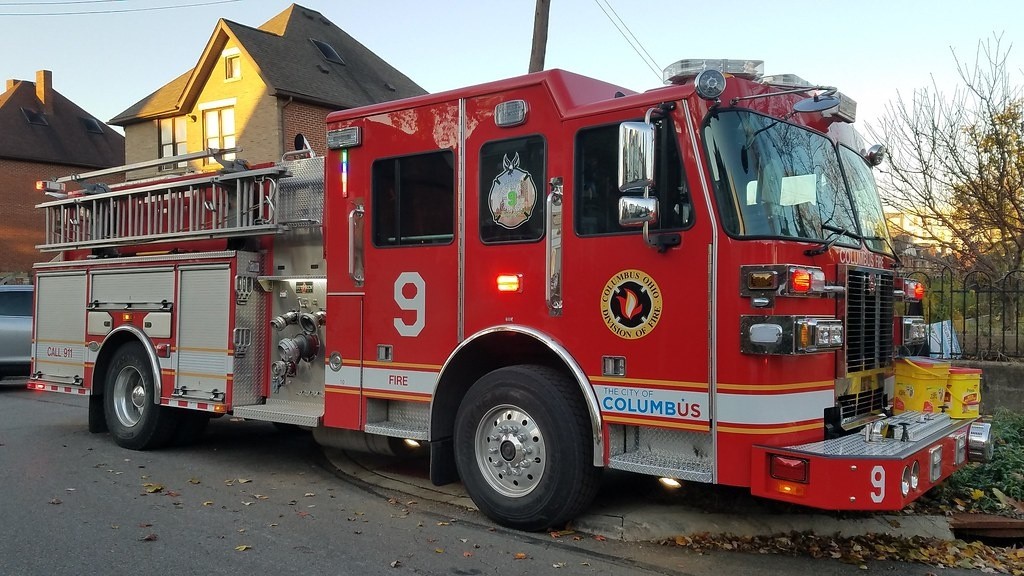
27,59 -> 994,532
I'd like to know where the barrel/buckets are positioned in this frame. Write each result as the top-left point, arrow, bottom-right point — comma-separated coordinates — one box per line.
893,356 -> 951,416
943,367 -> 983,419
